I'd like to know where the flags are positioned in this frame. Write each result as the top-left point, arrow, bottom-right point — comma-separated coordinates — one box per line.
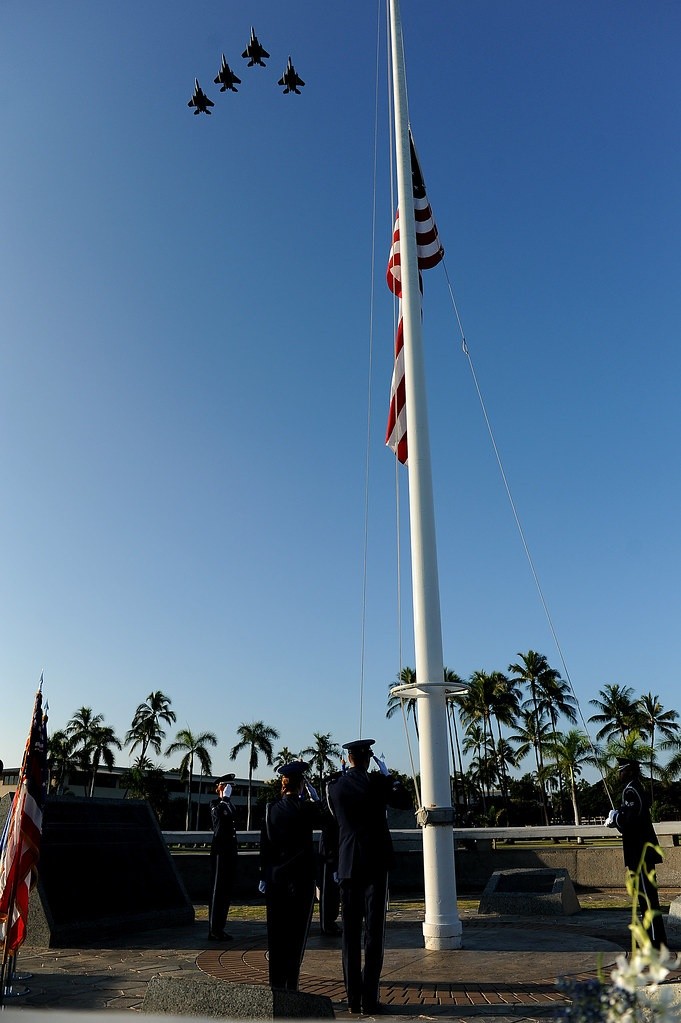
385,129 -> 445,467
0,692 -> 47,958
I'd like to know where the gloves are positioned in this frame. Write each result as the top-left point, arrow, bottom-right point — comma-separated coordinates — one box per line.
603,810 -> 619,827
333,872 -> 339,883
258,879 -> 266,894
373,756 -> 392,776
222,783 -> 233,798
304,778 -> 321,802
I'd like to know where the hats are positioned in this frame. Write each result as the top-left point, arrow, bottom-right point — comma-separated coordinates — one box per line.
214,774 -> 235,786
616,756 -> 640,772
342,739 -> 376,757
323,771 -> 344,784
278,761 -> 309,778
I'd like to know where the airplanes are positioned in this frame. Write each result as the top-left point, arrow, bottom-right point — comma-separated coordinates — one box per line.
213,53 -> 242,94
241,25 -> 271,67
277,55 -> 305,95
185,76 -> 214,118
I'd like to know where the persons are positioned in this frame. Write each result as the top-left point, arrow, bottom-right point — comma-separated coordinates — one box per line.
319,739 -> 413,1015
604,756 -> 667,949
257,762 -> 333,1018
208,774 -> 239,943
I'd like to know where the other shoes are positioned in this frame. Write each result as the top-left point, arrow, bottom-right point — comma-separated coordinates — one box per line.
320,922 -> 343,938
347,1003 -> 393,1015
208,931 -> 233,942
